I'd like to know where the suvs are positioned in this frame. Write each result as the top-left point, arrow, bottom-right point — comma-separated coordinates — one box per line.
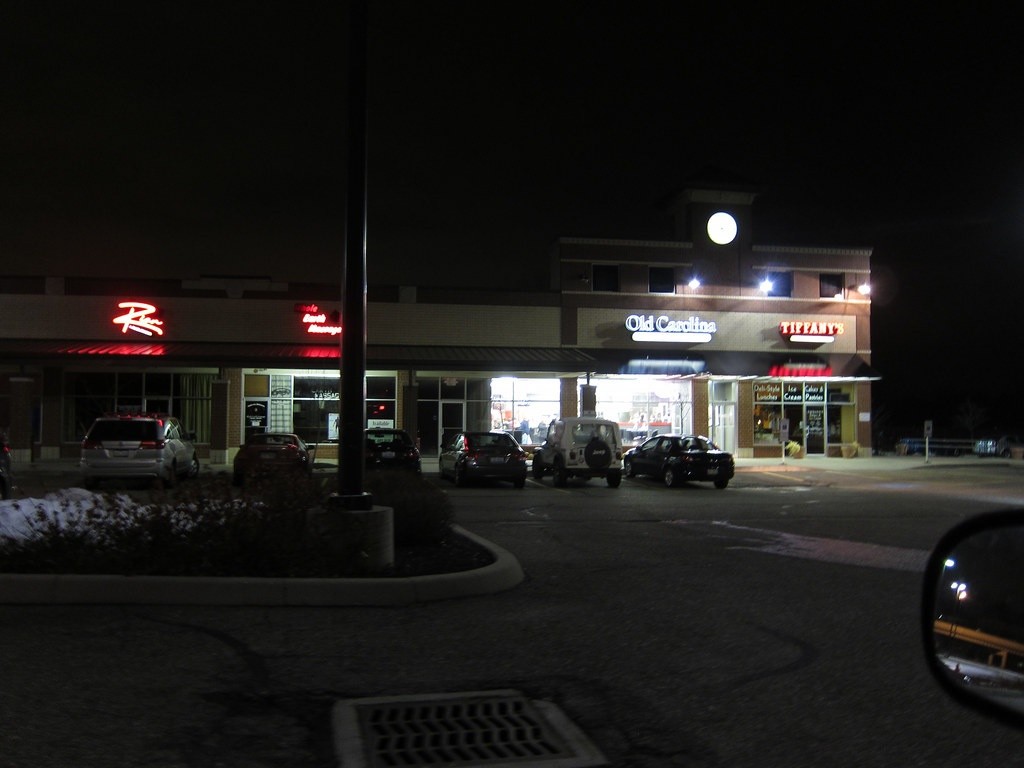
531,417 -> 623,489
78,411 -> 201,494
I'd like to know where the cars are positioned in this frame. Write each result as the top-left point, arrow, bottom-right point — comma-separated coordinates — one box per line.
362,425 -> 423,482
233,431 -> 314,496
438,432 -> 527,490
623,431 -> 735,490
973,433 -> 1020,458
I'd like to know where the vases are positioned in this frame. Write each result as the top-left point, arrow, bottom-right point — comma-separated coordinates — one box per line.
858,446 -> 872,456
826,443 -> 841,457
840,443 -> 857,458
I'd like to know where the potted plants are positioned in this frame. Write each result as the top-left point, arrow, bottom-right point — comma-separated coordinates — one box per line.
784,440 -> 804,459
